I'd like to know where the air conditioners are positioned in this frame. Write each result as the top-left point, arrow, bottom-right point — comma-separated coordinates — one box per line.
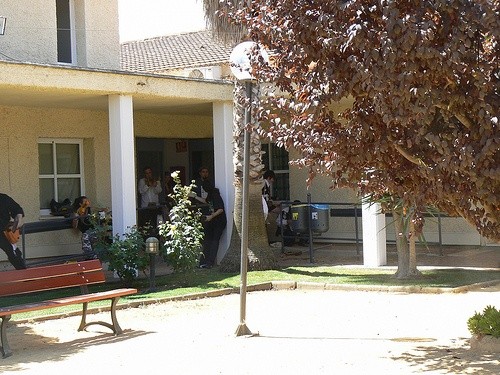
184,66 -> 222,79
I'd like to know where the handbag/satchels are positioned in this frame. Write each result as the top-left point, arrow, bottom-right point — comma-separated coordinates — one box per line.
50,199 -> 72,216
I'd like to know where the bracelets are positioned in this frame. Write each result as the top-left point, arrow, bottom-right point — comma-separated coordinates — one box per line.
194,195 -> 196,199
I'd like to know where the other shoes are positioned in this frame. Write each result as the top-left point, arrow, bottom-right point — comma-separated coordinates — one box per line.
299,241 -> 309,247
200,264 -> 212,269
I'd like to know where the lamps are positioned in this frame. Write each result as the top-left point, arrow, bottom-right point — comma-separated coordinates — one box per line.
145,237 -> 160,288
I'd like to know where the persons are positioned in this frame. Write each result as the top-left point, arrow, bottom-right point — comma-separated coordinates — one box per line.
139,166 -> 227,269
0,192 -> 36,296
262,171 -> 311,247
73,196 -> 110,260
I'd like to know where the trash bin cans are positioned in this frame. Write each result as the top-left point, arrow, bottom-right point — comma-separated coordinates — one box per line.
291,204 -> 308,234
311,204 -> 330,233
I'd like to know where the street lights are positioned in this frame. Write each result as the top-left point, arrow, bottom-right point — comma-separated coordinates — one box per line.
228,40 -> 269,336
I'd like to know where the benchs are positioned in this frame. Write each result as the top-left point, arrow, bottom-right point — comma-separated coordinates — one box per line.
20,219 -> 88,269
0,259 -> 137,359
